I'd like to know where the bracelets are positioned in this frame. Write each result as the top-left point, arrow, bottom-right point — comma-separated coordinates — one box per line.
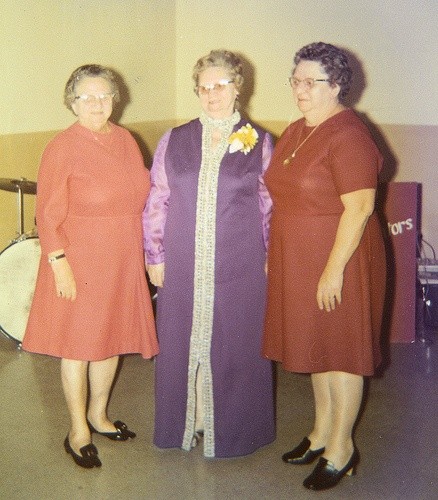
48,254 -> 65,263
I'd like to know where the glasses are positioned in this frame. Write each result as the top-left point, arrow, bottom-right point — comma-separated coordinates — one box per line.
288,77 -> 329,88
74,92 -> 116,107
192,79 -> 237,93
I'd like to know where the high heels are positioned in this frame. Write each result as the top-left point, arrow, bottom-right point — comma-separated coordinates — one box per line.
303,445 -> 359,490
281,437 -> 325,465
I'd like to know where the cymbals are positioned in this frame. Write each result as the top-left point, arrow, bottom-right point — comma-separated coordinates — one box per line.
0,176 -> 38,196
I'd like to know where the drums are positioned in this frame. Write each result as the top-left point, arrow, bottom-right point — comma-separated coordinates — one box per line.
0,229 -> 42,345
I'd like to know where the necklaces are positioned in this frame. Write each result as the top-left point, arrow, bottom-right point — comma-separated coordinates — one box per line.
283,122 -> 321,166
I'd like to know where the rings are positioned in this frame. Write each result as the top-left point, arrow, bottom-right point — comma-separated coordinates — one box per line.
329,297 -> 335,299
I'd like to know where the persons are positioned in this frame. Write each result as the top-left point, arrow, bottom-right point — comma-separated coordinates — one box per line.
261,42 -> 386,491
22,64 -> 160,468
142,50 -> 274,458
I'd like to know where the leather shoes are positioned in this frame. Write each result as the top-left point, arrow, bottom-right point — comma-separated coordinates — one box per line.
64,432 -> 103,468
87,414 -> 136,441
